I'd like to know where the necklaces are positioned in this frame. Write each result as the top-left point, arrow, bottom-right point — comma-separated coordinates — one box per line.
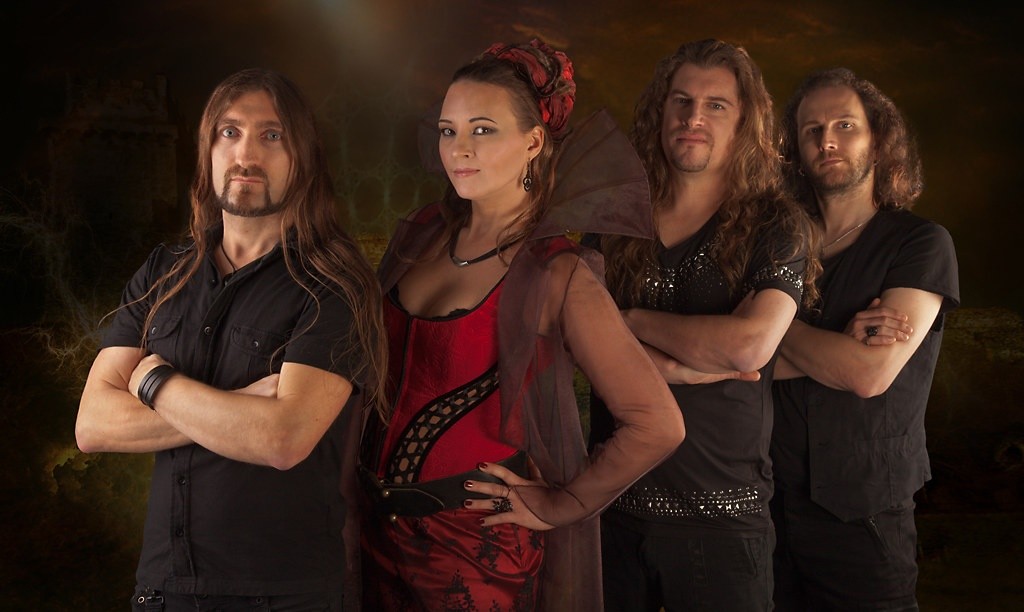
448,221 -> 523,267
220,240 -> 242,289
819,212 -> 875,252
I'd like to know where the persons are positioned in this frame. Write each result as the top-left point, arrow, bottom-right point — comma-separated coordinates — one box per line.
577,37 -> 823,611
773,64 -> 960,612
333,37 -> 688,612
77,66 -> 388,612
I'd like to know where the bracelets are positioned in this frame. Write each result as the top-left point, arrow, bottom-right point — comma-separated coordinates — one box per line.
136,364 -> 179,411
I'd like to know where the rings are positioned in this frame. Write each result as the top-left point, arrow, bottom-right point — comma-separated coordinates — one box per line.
491,497 -> 513,513
866,326 -> 879,336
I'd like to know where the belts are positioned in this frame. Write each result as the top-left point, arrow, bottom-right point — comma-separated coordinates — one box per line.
352,450 -> 529,519
